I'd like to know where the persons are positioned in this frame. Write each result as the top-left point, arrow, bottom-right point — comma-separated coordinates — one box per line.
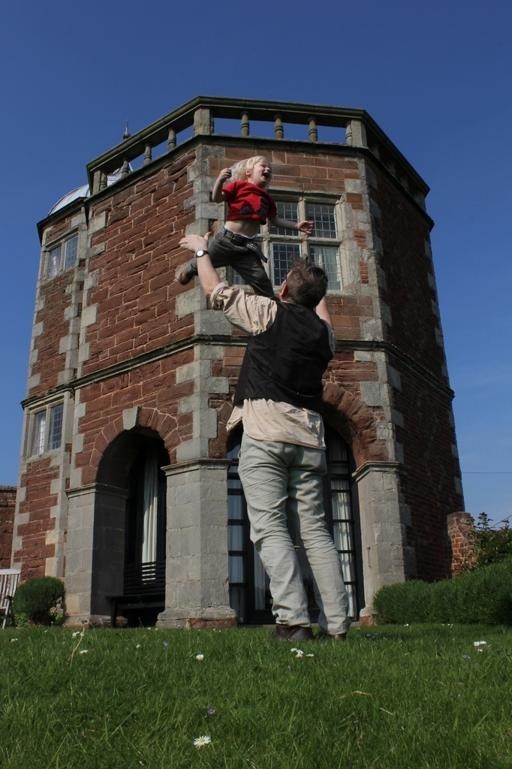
179,155 -> 314,304
177,226 -> 363,641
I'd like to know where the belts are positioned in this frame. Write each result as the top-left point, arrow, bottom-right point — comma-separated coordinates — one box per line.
219,226 -> 270,263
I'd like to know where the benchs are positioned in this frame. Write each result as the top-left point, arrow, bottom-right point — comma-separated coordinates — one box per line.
104,559 -> 166,628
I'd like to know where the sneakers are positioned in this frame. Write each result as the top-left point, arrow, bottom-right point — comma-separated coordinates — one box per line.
174,259 -> 197,287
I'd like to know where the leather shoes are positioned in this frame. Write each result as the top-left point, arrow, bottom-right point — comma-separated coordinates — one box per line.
266,624 -> 313,643
314,629 -> 349,644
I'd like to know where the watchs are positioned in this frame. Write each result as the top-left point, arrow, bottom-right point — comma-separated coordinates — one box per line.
194,251 -> 208,259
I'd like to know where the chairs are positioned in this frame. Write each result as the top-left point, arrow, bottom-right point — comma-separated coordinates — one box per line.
1,561 -> 22,632
